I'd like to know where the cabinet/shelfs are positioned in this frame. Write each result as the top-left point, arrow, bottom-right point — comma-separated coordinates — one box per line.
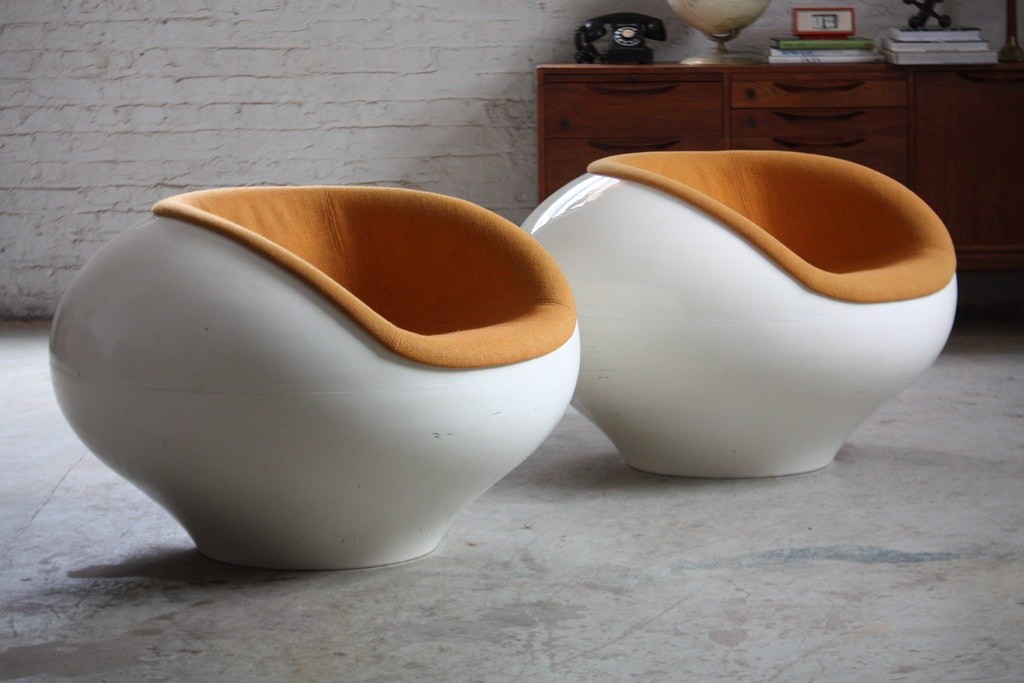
537,63 -> 1024,272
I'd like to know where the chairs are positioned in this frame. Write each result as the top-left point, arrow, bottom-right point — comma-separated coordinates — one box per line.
519,149 -> 958,480
49,185 -> 581,573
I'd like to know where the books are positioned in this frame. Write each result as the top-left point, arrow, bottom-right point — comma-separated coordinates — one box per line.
761,37 -> 884,64
880,27 -> 998,64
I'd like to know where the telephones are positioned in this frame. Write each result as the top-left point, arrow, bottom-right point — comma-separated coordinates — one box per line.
574,12 -> 667,64
